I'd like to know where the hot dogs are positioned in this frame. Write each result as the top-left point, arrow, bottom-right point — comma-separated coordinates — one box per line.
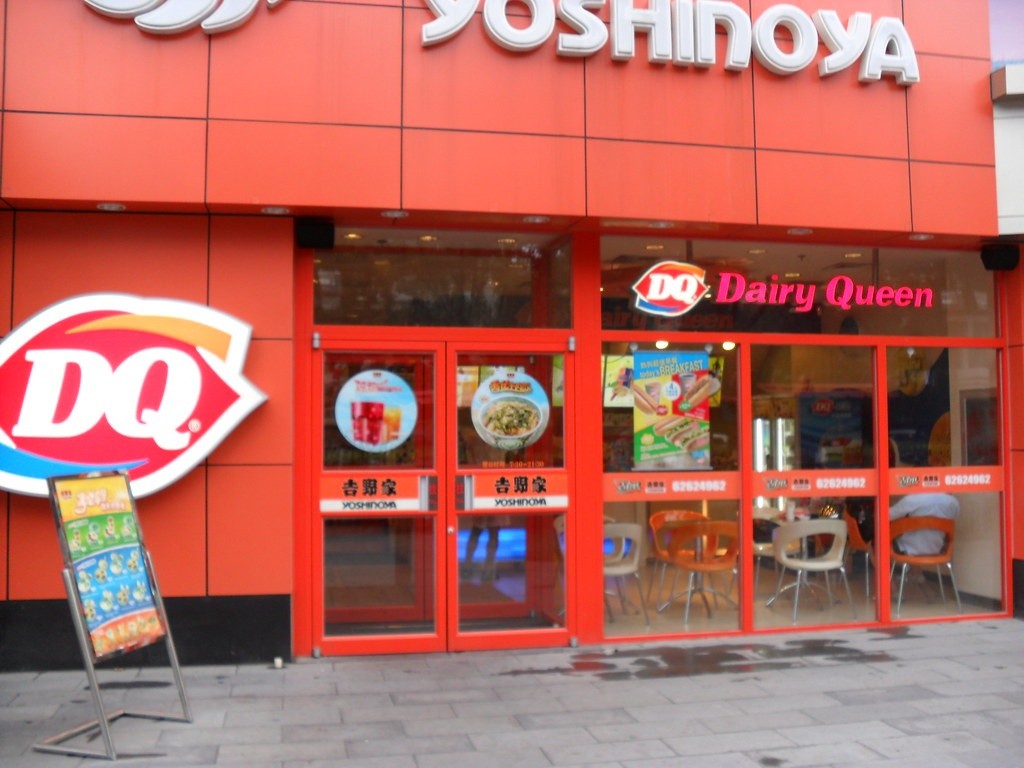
633,376 -> 720,450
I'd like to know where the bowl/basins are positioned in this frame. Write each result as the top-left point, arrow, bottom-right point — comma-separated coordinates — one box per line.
78,560 -> 146,620
479,396 -> 542,448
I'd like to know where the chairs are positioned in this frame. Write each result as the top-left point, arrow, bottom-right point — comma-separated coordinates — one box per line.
553,509 -> 962,625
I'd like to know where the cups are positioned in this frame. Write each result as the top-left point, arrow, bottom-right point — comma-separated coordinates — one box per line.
646,381 -> 660,405
379,407 -> 402,443
352,402 -> 383,444
681,374 -> 696,396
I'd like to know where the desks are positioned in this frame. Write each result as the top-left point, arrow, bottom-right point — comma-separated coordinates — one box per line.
717,541 -> 803,615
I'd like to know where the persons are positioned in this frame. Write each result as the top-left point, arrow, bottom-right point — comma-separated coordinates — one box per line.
890,494 -> 960,605
460,432 -> 508,580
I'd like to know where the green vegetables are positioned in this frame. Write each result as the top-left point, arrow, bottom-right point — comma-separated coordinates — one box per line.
491,406 -> 532,436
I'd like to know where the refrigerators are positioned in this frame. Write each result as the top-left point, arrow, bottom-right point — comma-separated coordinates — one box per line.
753,388 -> 870,569
